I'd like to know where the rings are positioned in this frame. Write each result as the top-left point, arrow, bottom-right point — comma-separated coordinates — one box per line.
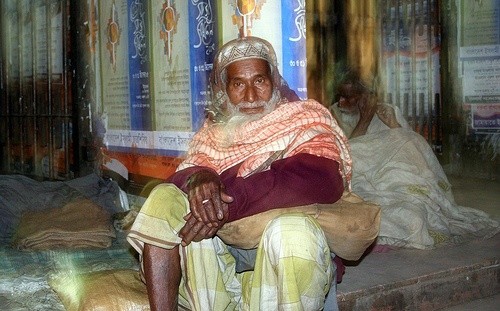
202,198 -> 209,204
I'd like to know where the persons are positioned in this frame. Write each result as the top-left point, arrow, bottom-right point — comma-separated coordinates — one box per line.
125,36 -> 352,311
327,68 -> 431,249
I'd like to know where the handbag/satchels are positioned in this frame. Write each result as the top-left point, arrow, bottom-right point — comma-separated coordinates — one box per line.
215,148 -> 381,261
